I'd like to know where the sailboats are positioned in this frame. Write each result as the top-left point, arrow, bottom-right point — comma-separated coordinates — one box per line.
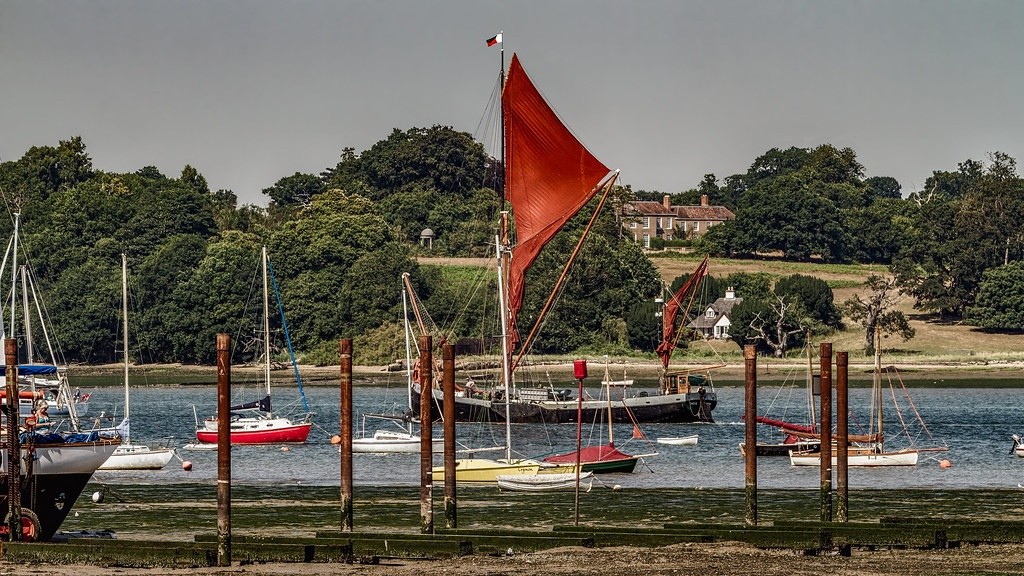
340,270 -> 461,454
419,30 -> 741,493
198,245 -> 315,443
738,332 -> 866,458
0,197 -> 177,542
786,322 -> 948,466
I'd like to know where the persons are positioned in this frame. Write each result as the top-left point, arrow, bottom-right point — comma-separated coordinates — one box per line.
34,401 -> 57,436
